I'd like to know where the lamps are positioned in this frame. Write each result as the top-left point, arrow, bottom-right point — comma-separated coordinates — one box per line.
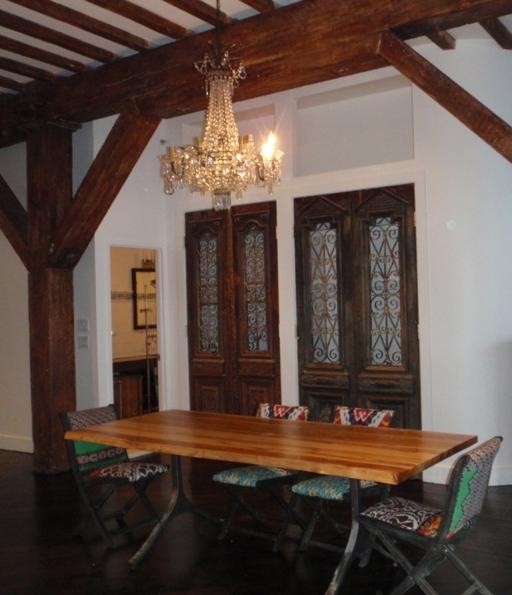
157,0 -> 284,213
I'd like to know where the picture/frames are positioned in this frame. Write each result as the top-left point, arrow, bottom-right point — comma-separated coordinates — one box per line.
131,267 -> 157,330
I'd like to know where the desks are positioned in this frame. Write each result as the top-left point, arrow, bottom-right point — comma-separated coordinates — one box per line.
64,409 -> 478,595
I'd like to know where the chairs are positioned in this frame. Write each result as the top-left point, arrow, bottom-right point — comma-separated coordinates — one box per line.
57,403 -> 169,552
355,435 -> 503,595
291,405 -> 399,567
213,402 -> 311,554
